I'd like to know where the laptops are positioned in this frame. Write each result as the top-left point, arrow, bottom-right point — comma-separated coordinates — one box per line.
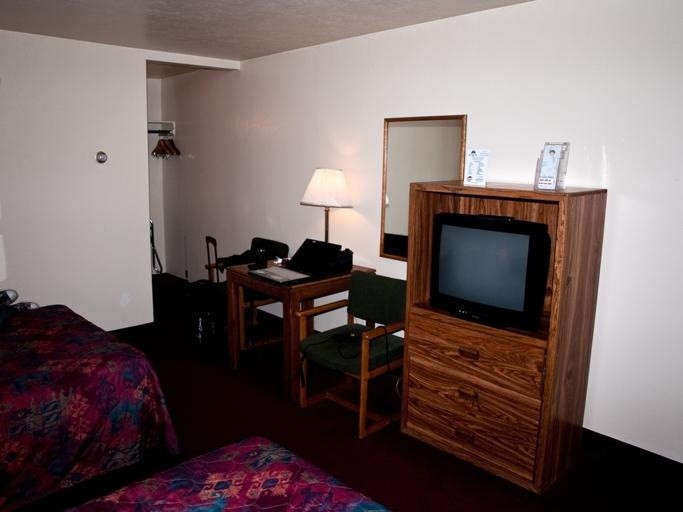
249,237 -> 342,287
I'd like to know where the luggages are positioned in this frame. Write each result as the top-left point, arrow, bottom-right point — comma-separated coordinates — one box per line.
176,237 -> 231,365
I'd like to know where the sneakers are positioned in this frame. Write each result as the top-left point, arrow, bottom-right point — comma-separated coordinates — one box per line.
9,302 -> 39,312
0,290 -> 18,306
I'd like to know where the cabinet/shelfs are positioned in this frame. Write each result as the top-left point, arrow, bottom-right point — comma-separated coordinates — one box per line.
399,178 -> 611,487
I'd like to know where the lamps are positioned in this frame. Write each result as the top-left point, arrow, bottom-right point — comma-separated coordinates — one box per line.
299,167 -> 354,240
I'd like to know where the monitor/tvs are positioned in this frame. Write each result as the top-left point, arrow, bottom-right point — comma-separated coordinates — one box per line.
428,211 -> 552,333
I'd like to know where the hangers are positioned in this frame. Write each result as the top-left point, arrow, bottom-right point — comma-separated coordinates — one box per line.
152,130 -> 181,160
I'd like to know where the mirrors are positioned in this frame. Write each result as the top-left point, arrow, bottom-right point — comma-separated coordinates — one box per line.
377,114 -> 468,259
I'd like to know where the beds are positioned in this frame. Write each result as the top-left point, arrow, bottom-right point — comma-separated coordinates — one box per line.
0,300 -> 169,512
69,436 -> 389,512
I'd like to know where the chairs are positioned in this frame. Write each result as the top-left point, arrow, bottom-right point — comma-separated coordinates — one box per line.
295,269 -> 407,438
213,240 -> 289,329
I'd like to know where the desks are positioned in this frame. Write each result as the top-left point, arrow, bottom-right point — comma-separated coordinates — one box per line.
224,256 -> 375,403
